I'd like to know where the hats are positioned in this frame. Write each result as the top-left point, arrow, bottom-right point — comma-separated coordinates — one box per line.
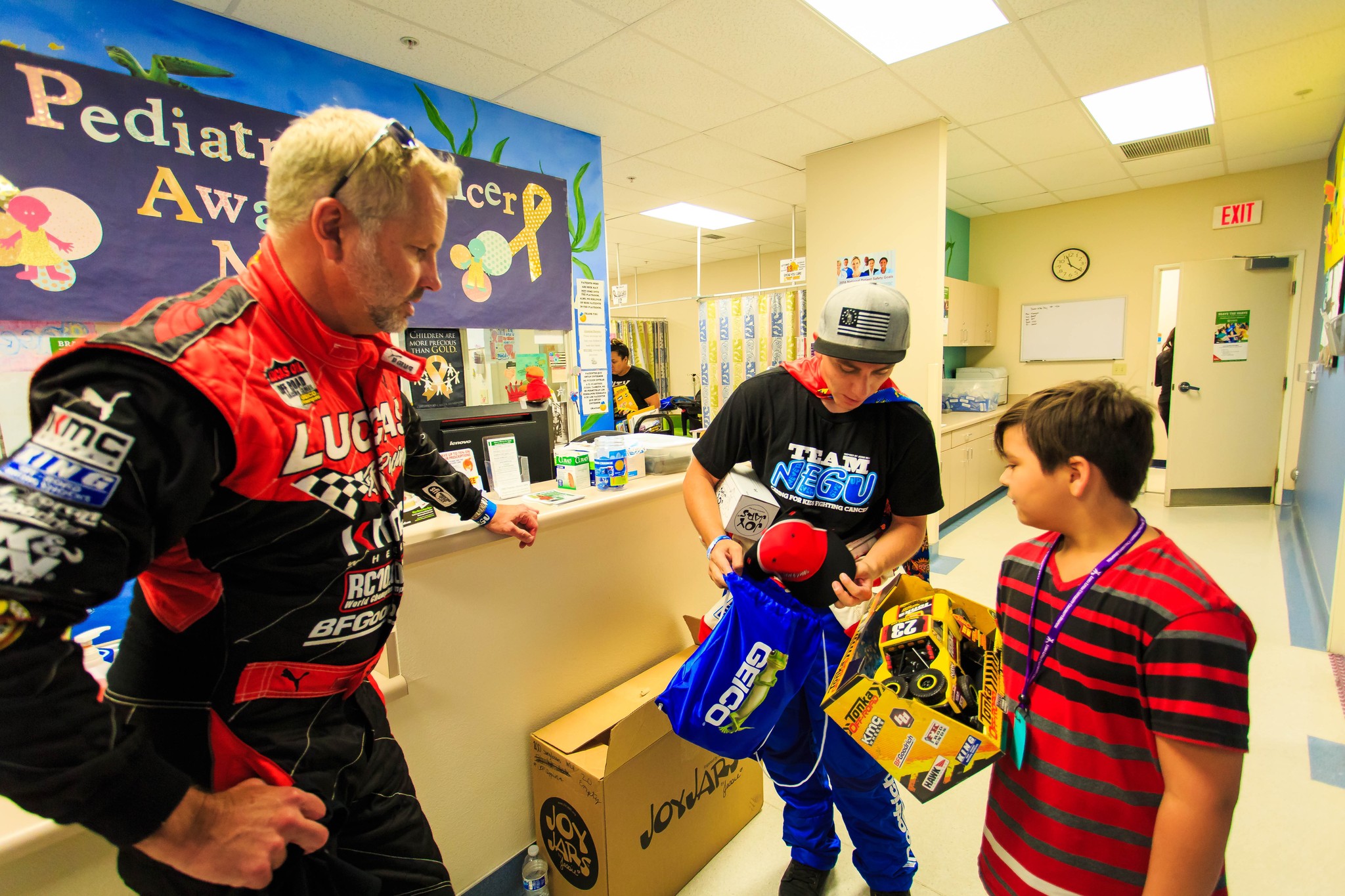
812,280 -> 910,365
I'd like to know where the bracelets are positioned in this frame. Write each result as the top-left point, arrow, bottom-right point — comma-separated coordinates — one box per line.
706,535 -> 732,561
471,495 -> 497,527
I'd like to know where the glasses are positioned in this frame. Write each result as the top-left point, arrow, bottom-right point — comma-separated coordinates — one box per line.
327,116 -> 415,203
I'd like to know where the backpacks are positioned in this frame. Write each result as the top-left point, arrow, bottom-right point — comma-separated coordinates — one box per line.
653,565 -> 832,788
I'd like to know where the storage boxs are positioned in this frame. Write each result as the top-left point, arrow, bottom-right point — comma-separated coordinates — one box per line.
613,433 -> 702,475
715,464 -> 780,542
554,451 -> 590,490
623,440 -> 645,480
529,642 -> 764,896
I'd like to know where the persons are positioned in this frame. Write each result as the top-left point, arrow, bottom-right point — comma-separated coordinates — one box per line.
1154,326 -> 1175,439
1214,322 -> 1249,343
837,256 -> 893,279
977,376 -> 1255,896
679,283 -> 943,896
0,100 -> 547,896
610,338 -> 660,419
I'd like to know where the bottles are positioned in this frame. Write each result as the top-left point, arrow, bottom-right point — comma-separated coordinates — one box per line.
522,845 -> 549,896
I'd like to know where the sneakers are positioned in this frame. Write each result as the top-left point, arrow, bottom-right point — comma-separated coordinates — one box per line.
778,856 -> 831,896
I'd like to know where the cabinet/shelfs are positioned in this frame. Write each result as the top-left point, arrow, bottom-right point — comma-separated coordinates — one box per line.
938,400 -> 1015,533
974,284 -> 999,345
948,276 -> 974,347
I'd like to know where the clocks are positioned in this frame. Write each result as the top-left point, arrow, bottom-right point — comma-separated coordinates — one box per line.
1052,247 -> 1090,282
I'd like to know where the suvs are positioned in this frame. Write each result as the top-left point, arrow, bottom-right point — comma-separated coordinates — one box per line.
874,594 -> 982,724
